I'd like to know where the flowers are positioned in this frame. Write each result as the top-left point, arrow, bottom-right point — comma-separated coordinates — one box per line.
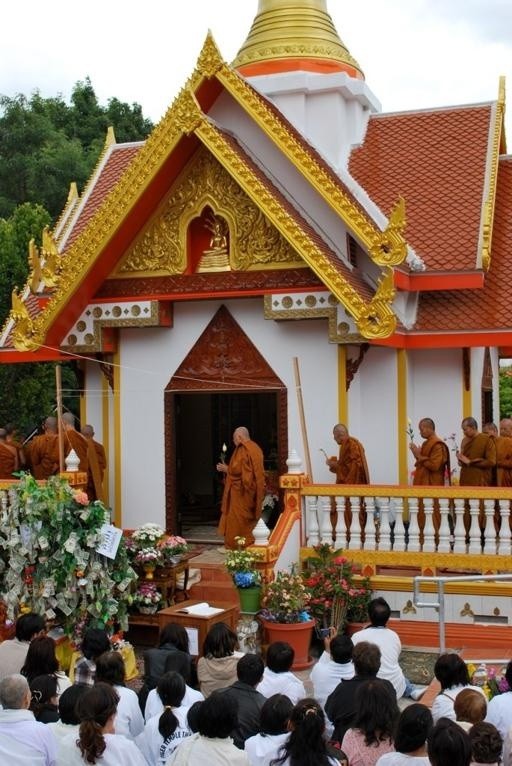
262,559 -> 313,619
316,447 -> 329,460
406,419 -> 414,441
220,444 -> 227,465
123,524 -> 187,614
301,543 -> 374,622
224,536 -> 261,587
443,431 -> 460,452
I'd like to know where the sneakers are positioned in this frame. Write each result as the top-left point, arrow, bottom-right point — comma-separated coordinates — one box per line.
216,545 -> 246,554
410,686 -> 430,701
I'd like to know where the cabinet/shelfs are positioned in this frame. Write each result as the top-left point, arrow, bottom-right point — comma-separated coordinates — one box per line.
126,562 -> 189,645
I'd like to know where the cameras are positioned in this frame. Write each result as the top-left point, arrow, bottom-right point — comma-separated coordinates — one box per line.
321,629 -> 331,637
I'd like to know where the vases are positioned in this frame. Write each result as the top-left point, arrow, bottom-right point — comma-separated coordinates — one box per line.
265,617 -> 316,666
348,621 -> 371,635
239,588 -> 260,612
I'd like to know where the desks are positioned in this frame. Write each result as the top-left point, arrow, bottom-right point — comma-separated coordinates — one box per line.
158,597 -> 240,667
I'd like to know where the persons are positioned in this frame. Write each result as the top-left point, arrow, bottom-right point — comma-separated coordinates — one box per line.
455,414 -> 512,546
202,223 -> 228,256
0,411 -> 107,501
216,426 -> 265,553
409,418 -> 451,549
326,424 -> 371,542
0,598 -> 511,766
